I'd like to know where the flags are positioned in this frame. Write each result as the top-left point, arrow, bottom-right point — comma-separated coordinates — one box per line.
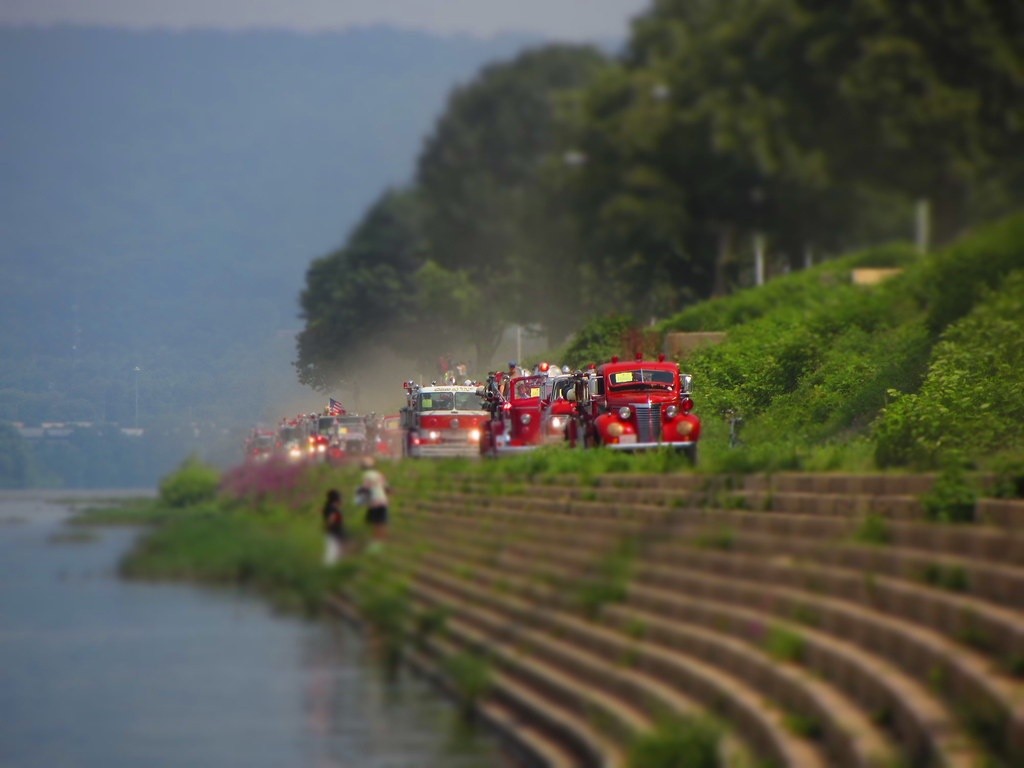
329,398 -> 343,415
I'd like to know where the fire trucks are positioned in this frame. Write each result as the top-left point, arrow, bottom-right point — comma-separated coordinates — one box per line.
564,352 -> 701,468
474,361 -> 595,451
241,411 -> 402,472
398,376 -> 493,459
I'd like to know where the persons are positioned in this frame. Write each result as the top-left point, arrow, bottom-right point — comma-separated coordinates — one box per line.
444,361 -> 467,383
517,384 -> 531,398
355,455 -> 392,538
321,489 -> 346,569
508,360 -> 516,374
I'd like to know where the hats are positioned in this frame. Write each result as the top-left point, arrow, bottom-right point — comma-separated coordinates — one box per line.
361,456 -> 374,467
508,360 -> 516,366
